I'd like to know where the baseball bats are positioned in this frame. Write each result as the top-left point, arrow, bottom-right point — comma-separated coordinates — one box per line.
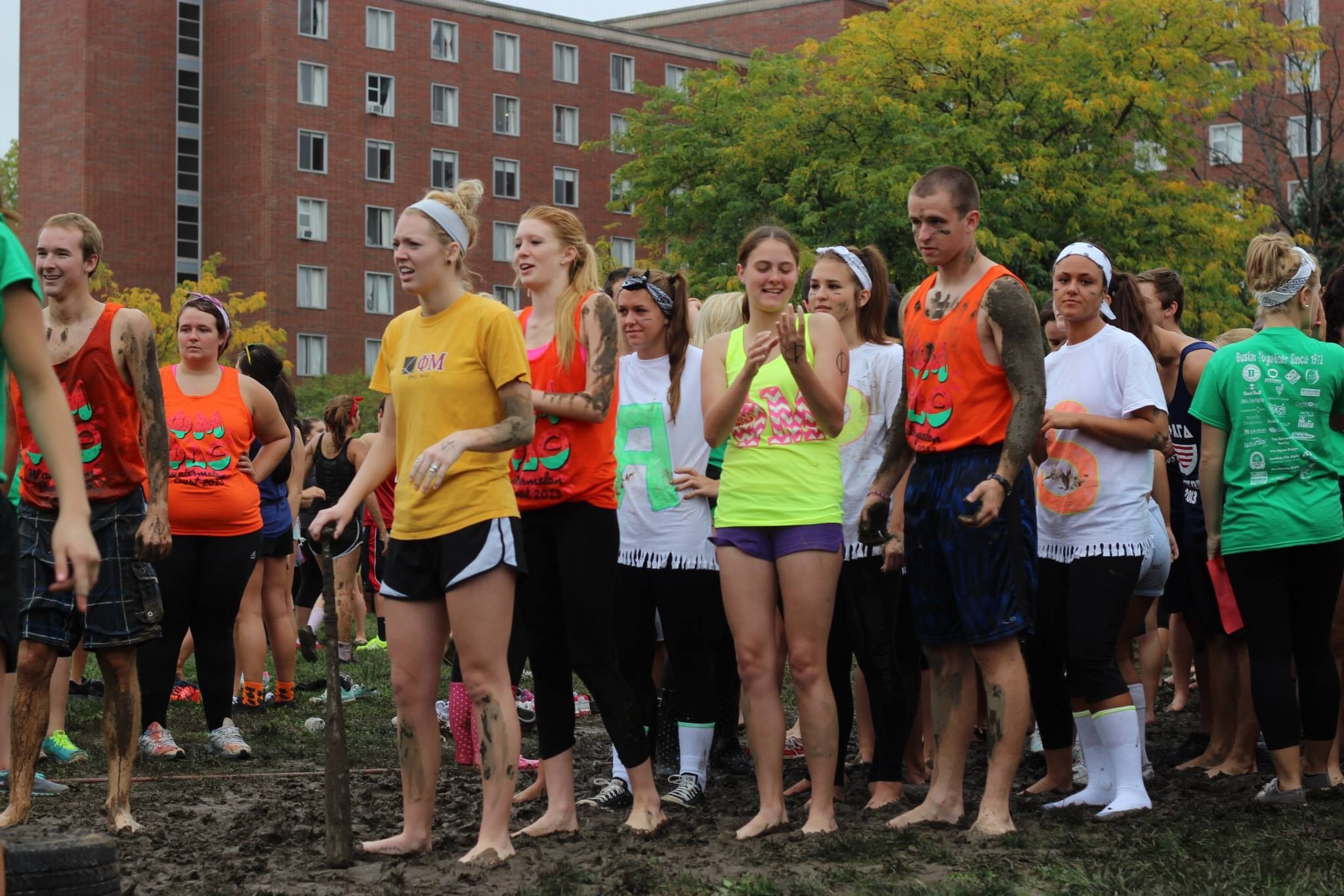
318,527 -> 354,867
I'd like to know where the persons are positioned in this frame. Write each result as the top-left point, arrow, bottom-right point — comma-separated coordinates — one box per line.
307,180 -> 538,864
0,164 -> 1344,841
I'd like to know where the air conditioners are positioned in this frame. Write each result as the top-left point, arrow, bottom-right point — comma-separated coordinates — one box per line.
302,229 -> 316,240
1208,152 -> 1224,167
369,104 -> 382,114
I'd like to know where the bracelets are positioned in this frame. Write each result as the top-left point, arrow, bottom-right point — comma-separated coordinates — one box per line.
985,473 -> 1014,500
866,490 -> 891,502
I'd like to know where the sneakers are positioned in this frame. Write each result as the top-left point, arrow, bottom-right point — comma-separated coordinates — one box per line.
308,684 -> 356,705
204,717 -> 252,757
69,676 -> 105,698
138,722 -> 186,760
660,771 -> 709,811
42,729 -> 90,763
170,681 -> 201,704
353,682 -> 379,698
575,777 -> 634,812
572,691 -> 590,719
356,635 -> 387,652
0,768 -> 70,797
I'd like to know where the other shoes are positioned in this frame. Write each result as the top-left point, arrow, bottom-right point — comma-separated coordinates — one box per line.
297,624 -> 318,663
352,637 -> 370,645
1072,744 -> 1090,786
392,700 -> 452,735
1253,771 -> 1332,805
516,686 -> 535,722
1029,720 -> 1044,752
338,653 -> 360,665
232,695 -> 298,715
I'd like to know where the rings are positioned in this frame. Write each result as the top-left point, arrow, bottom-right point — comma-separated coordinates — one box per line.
429,463 -> 439,473
